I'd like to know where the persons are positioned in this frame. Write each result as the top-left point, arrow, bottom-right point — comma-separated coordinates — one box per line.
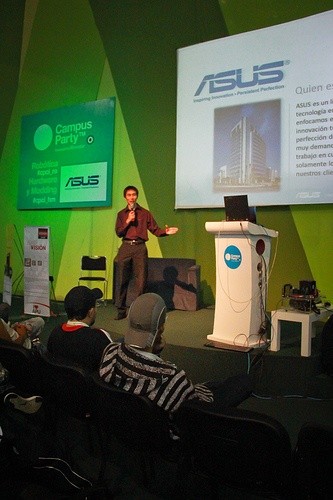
99,293 -> 215,440
46,286 -> 112,376
0,365 -> 45,414
0,316 -> 45,348
114,186 -> 179,320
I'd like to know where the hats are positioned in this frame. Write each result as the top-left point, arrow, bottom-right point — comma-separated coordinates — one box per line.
124,293 -> 167,349
64,286 -> 103,313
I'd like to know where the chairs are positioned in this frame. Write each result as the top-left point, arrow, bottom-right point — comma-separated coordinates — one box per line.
0,342 -> 333,500
79,254 -> 108,305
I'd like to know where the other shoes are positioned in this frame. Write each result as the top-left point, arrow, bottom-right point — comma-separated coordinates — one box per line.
4,393 -> 42,414
115,313 -> 127,320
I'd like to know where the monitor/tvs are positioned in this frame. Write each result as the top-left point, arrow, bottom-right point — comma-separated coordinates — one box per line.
224,195 -> 250,222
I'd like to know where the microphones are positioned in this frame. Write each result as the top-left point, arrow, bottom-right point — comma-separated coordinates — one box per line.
130,202 -> 136,226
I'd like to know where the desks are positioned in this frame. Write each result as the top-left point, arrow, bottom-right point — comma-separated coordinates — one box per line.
272,304 -> 333,356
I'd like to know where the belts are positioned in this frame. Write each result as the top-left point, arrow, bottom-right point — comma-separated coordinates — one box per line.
123,240 -> 144,246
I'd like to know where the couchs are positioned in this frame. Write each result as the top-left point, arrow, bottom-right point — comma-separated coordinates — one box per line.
113,257 -> 200,313
